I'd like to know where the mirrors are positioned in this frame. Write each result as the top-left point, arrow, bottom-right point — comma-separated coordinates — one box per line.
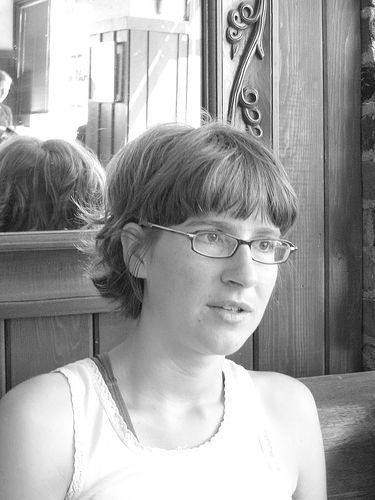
0,0 -> 275,319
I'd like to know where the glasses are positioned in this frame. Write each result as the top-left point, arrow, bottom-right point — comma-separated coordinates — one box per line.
133,216 -> 298,264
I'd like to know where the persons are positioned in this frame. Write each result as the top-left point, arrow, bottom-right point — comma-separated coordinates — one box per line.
0,123 -> 326,500
0,136 -> 110,232
0,69 -> 16,143
